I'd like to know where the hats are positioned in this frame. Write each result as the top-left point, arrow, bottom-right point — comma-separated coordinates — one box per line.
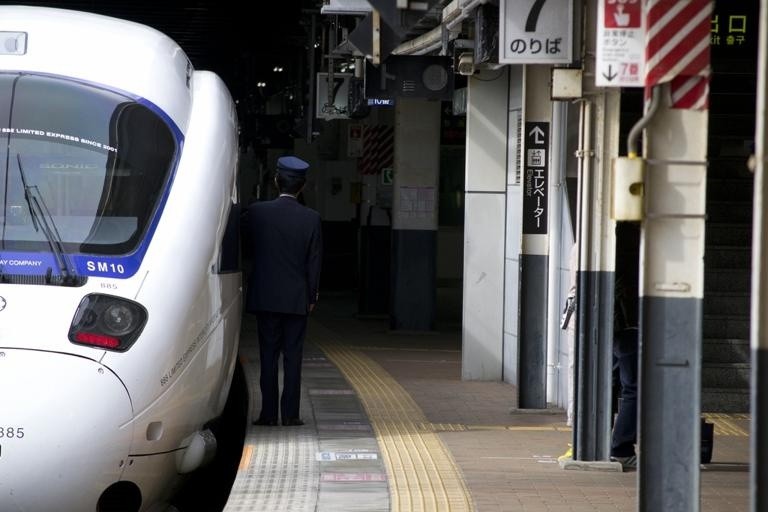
275,156 -> 310,177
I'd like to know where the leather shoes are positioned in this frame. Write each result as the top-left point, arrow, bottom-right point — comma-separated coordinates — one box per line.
251,417 -> 305,427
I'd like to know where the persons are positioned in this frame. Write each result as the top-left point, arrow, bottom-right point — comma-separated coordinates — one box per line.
237,157 -> 325,426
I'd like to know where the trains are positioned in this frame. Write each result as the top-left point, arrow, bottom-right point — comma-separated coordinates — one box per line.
1,4 -> 247,512
